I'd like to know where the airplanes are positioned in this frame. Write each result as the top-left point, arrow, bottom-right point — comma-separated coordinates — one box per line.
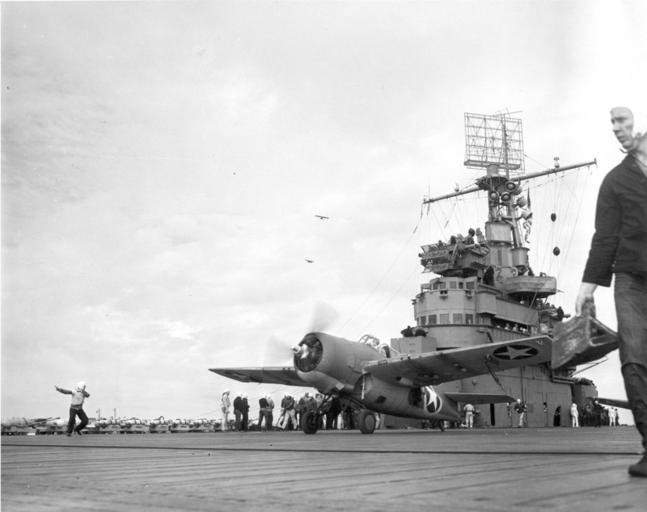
209,331 -> 556,434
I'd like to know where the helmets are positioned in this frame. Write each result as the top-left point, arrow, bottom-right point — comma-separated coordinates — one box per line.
609,98 -> 646,138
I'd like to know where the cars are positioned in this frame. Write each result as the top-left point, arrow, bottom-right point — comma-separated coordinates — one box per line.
88,420 -> 227,432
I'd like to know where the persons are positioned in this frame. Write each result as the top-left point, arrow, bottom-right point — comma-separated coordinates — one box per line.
463,403 -> 475,428
570,399 -> 619,428
575,107 -> 647,477
54,382 -> 90,436
514,398 -> 527,428
554,406 -> 560,426
220,388 -> 338,430
